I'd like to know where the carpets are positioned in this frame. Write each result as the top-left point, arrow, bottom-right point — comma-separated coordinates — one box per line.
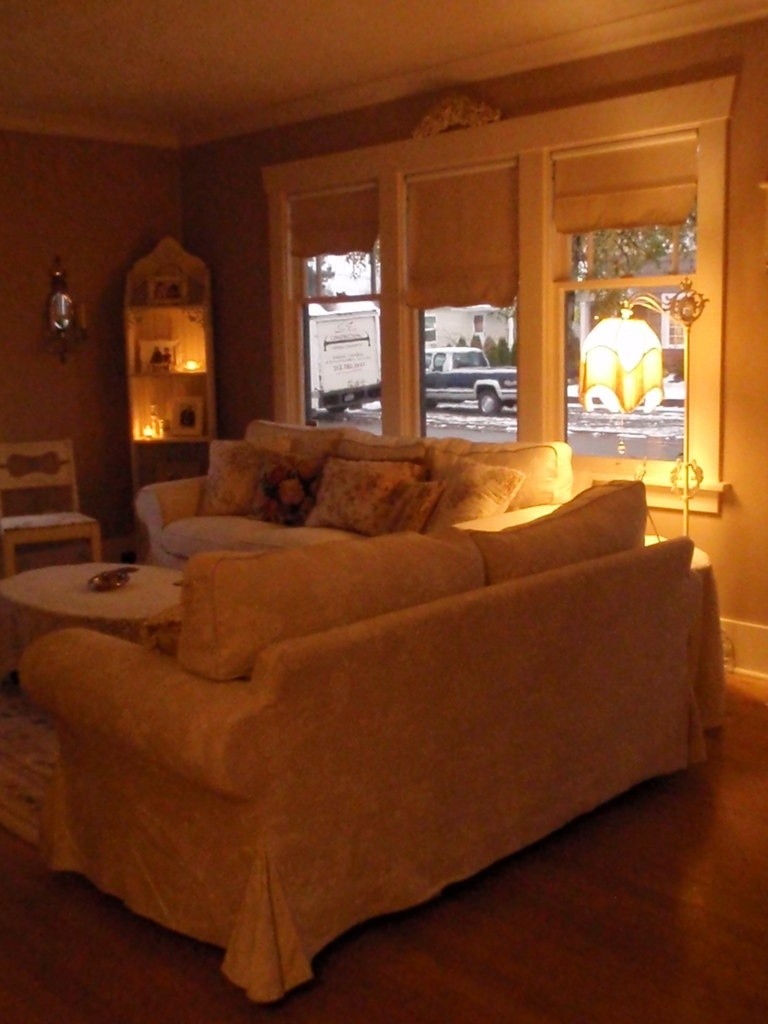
0,680 -> 56,844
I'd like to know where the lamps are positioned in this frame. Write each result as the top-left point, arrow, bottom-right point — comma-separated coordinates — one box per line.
578,278 -> 709,537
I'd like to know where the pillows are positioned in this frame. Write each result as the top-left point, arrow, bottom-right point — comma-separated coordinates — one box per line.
468,480 -> 644,584
197,438 -> 291,514
333,438 -> 427,462
247,450 -> 327,527
303,457 -> 425,531
179,523 -> 487,681
352,472 -> 447,537
419,452 -> 526,534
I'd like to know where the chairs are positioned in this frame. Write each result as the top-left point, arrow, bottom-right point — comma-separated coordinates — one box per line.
0,437 -> 102,576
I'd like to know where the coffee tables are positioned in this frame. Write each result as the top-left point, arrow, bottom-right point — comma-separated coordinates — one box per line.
0,561 -> 181,654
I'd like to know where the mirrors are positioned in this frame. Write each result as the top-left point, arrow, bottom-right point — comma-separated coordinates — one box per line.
50,252 -> 77,340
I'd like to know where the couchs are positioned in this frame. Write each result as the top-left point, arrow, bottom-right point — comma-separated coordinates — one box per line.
131,418 -> 575,569
20,481 -> 711,1003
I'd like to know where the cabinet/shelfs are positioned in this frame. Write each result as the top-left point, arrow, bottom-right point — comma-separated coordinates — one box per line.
123,234 -> 217,491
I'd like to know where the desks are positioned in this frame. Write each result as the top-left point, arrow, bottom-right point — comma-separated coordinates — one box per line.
646,534 -> 726,731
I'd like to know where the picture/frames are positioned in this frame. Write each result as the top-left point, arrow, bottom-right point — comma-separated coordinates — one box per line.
170,396 -> 204,435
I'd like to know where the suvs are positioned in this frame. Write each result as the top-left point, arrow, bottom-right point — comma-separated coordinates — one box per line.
421,347 -> 518,416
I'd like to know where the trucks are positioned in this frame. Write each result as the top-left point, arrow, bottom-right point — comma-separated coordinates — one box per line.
311,300 -> 384,416
